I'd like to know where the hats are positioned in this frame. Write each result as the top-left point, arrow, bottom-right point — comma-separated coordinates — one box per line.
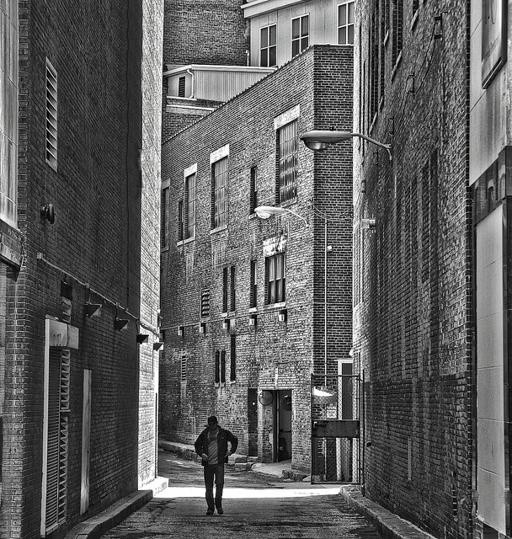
208,416 -> 217,424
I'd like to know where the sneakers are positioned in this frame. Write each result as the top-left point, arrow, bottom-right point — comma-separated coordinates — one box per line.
206,507 -> 214,516
216,505 -> 224,514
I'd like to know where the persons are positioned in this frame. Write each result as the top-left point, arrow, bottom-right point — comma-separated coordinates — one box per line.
194,415 -> 238,516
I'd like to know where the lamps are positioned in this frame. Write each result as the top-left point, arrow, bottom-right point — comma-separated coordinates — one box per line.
254,206 -> 309,228
300,130 -> 392,162
